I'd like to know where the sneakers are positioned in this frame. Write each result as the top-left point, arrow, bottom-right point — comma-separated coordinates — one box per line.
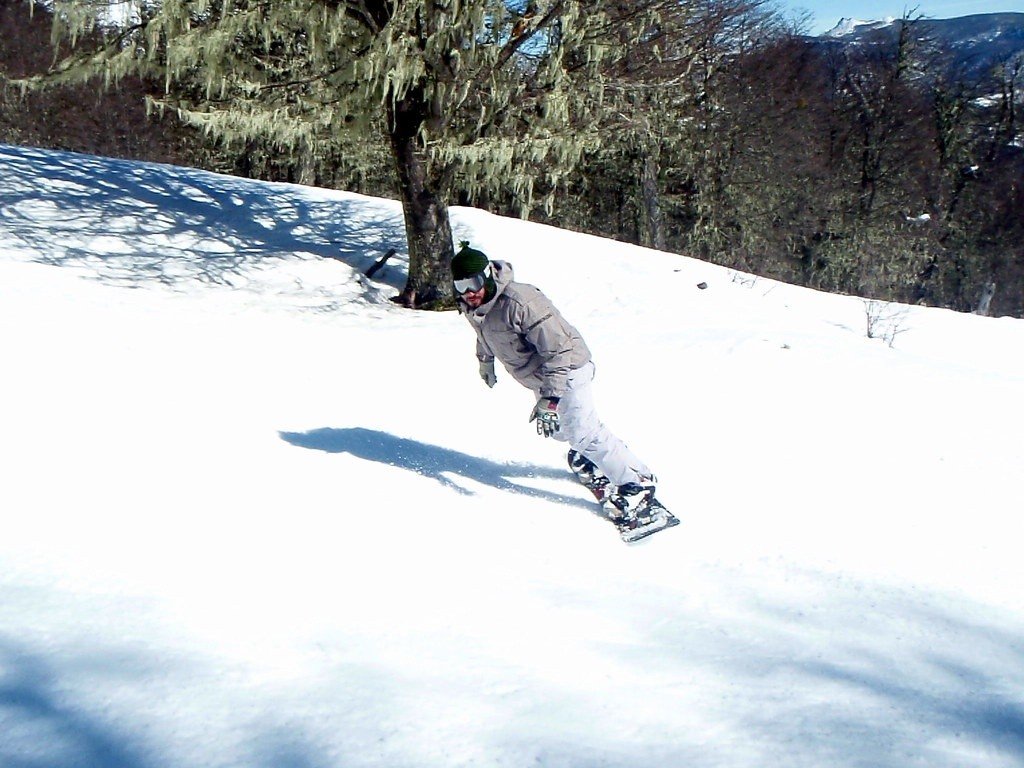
603,480 -> 656,519
571,455 -> 590,472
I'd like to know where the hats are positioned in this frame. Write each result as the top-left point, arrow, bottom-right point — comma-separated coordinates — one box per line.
450,239 -> 497,315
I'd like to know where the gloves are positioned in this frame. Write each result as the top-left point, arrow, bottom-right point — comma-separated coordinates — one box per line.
479,361 -> 497,388
529,396 -> 562,438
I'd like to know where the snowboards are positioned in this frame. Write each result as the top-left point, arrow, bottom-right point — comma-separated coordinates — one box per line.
567,445 -> 681,544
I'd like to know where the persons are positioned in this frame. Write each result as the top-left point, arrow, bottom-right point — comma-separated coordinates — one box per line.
451,242 -> 656,518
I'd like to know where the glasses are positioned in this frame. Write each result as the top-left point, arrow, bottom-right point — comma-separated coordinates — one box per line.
453,264 -> 492,295
